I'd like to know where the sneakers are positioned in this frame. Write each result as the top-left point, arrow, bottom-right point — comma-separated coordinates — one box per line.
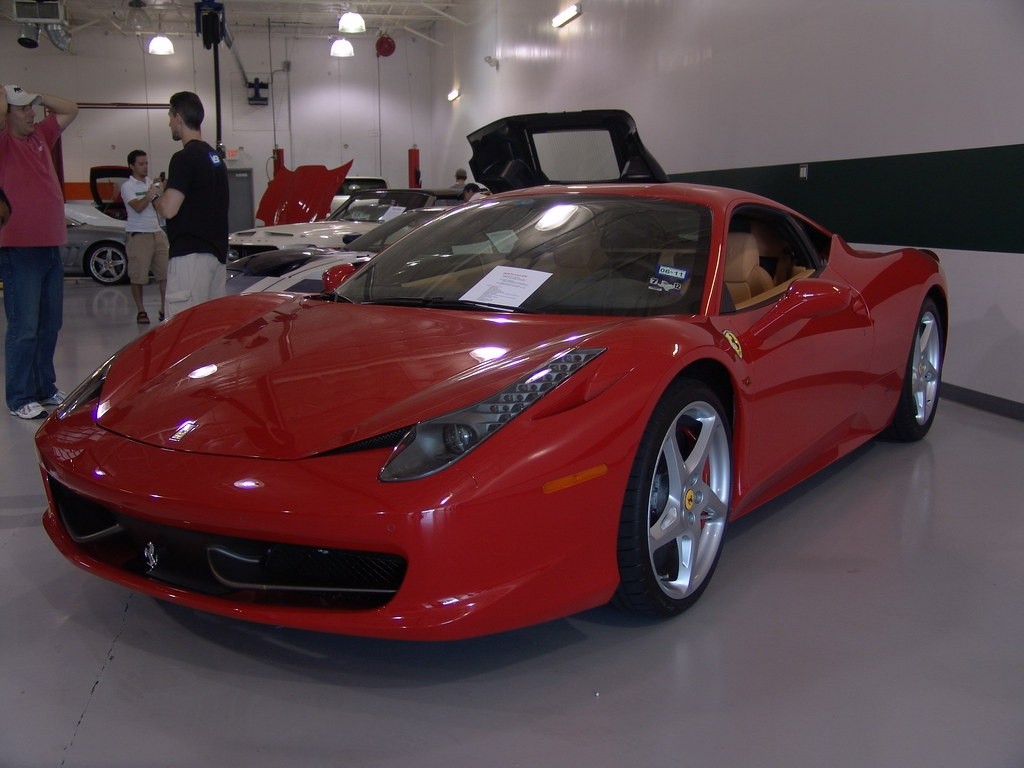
9,402 -> 48,419
37,389 -> 68,406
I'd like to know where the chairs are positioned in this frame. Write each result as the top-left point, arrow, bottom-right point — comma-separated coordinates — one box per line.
522,219 -> 608,311
658,234 -> 775,316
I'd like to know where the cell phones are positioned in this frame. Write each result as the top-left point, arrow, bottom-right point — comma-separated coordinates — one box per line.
160,171 -> 166,182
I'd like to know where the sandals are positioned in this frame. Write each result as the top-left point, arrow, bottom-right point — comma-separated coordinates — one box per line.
158,310 -> 164,321
137,311 -> 150,324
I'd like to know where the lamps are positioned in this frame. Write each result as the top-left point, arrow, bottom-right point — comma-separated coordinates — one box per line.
338,11 -> 366,33
147,34 -> 175,55
551,3 -> 581,28
330,39 -> 354,57
447,88 -> 462,102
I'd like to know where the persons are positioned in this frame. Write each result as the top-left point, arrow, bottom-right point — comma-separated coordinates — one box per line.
448,169 -> 467,206
464,183 -> 486,201
120,148 -> 170,325
0,84 -> 79,419
147,91 -> 230,321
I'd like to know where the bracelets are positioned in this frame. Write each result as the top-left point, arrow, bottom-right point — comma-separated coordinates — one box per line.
151,196 -> 158,203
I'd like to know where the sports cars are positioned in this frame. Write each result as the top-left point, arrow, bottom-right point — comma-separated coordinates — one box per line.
31,187 -> 950,639
227,108 -> 671,297
61,166 -> 157,285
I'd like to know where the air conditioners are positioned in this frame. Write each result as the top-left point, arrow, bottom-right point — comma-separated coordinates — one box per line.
13,0 -> 71,27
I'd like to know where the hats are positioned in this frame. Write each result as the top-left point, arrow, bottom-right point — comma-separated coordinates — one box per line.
454,168 -> 467,177
4,85 -> 43,106
